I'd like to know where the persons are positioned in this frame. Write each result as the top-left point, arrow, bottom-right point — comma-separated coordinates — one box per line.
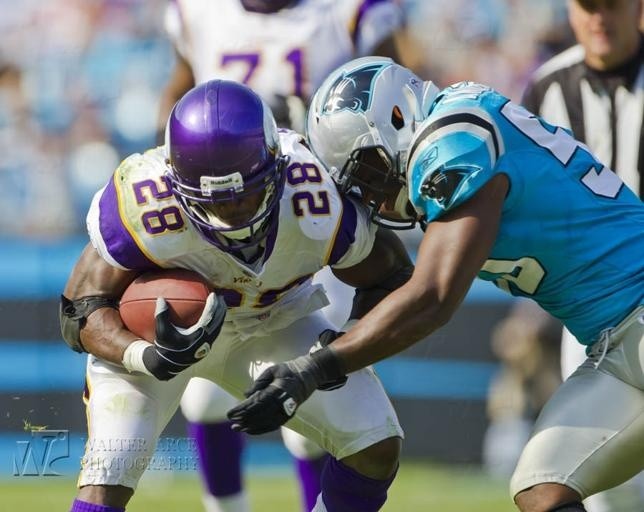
490,0 -> 644,512
226,56 -> 644,512
59,77 -> 415,512
1,0 -> 577,238
154,0 -> 408,512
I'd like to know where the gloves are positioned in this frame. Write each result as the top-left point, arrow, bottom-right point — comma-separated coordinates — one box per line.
143,293 -> 227,380
226,327 -> 353,435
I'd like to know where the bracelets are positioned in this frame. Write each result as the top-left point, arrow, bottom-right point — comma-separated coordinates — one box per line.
123,340 -> 155,378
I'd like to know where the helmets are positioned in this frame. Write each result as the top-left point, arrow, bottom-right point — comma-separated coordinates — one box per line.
306,55 -> 440,229
163,79 -> 287,251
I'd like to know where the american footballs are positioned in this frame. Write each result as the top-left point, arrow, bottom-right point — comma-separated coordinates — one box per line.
117,267 -> 212,347
